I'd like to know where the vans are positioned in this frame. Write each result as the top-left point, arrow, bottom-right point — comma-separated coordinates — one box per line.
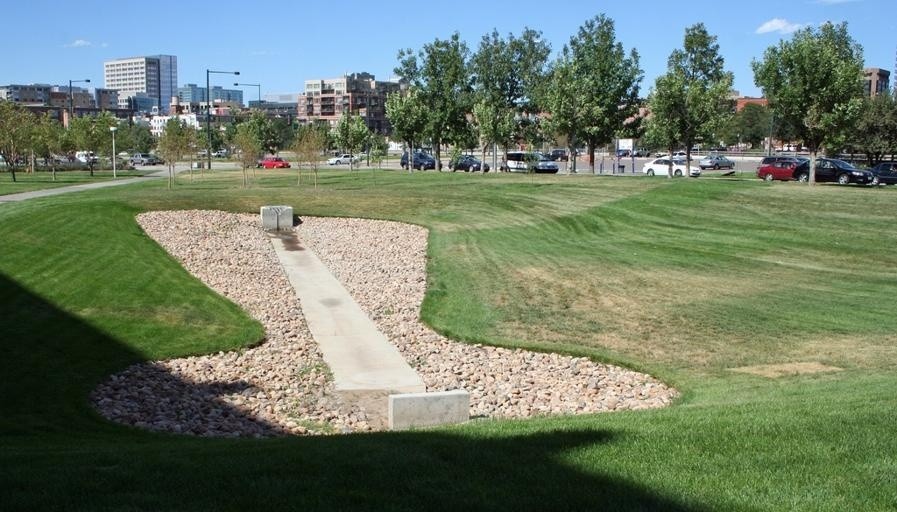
498,151 -> 561,175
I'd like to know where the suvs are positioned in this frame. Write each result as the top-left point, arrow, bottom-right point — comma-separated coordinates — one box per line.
868,159 -> 897,187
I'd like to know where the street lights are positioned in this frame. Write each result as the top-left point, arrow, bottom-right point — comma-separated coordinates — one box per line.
70,77 -> 90,118
737,133 -> 740,151
231,79 -> 261,112
108,126 -> 119,179
206,69 -> 241,170
306,96 -> 312,123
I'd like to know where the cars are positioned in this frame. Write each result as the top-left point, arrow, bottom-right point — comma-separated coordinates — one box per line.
196,147 -> 209,158
755,154 -> 817,185
642,158 -> 702,177
774,142 -> 807,151
325,153 -> 360,166
790,157 -> 875,186
397,149 -> 444,172
696,150 -> 737,171
446,153 -> 490,174
261,156 -> 292,170
0,148 -> 165,169
210,147 -> 226,158
619,139 -> 728,166
542,148 -> 569,161
255,158 -> 263,167
